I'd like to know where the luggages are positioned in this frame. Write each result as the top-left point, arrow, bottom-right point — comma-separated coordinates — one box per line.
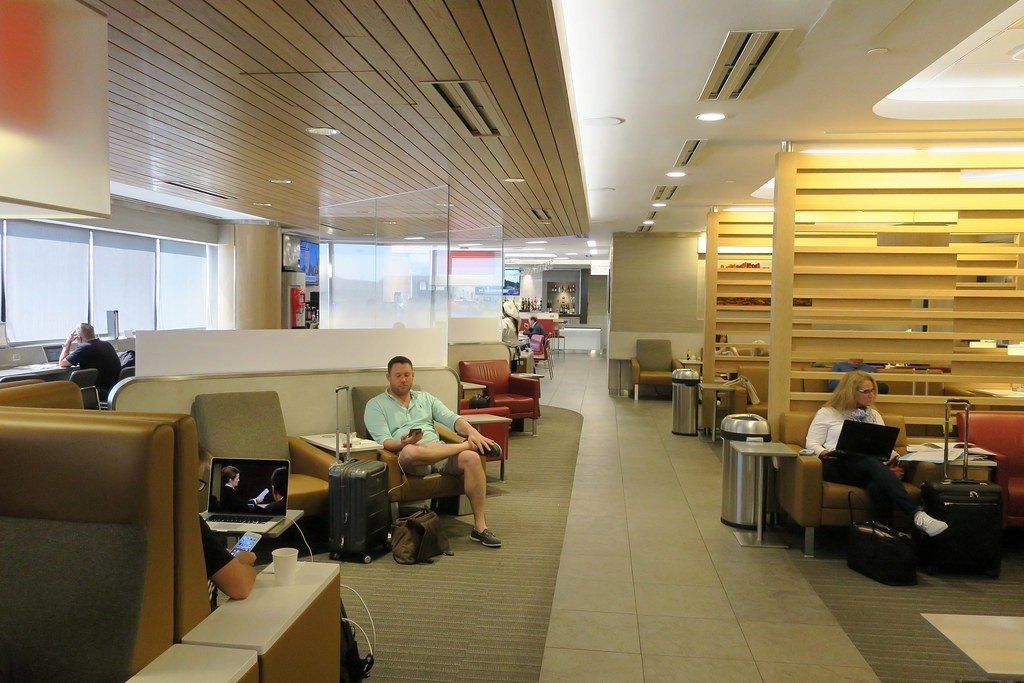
923,399 -> 1002,580
328,385 -> 391,564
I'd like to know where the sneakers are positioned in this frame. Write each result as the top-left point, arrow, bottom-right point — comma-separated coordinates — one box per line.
463,439 -> 502,457
470,528 -> 501,546
914,512 -> 949,538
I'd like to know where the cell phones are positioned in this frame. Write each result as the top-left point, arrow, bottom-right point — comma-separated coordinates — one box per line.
798,449 -> 814,456
230,530 -> 262,559
259,488 -> 269,498
409,428 -> 421,439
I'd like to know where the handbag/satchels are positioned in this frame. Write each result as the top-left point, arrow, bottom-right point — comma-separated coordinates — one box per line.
848,490 -> 918,587
391,509 -> 449,565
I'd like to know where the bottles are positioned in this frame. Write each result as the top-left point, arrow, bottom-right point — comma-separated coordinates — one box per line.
305,304 -> 312,320
551,283 -> 575,293
687,348 -> 690,359
504,297 -> 542,311
556,297 -> 575,314
547,299 -> 552,312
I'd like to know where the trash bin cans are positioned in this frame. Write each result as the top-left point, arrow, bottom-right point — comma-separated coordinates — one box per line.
720,413 -> 770,530
671,368 -> 700,436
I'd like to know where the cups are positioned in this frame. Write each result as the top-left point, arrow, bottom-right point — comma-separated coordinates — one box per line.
727,373 -> 738,380
343,432 -> 357,447
271,548 -> 299,585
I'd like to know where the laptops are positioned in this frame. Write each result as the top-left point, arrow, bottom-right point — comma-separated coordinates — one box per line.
203,457 -> 290,534
826,420 -> 900,462
41,344 -> 64,364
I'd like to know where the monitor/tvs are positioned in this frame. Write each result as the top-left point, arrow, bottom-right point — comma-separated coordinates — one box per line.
503,269 -> 521,295
299,240 -> 319,286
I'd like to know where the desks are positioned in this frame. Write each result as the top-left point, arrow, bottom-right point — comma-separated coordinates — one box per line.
300,432 -> 384,462
459,413 -> 512,481
678,358 -> 704,375
609,357 -> 634,399
459,381 -> 486,400
948,460 -> 997,483
729,439 -> 797,549
516,351 -> 534,374
699,382 -> 734,442
0,362 -> 79,379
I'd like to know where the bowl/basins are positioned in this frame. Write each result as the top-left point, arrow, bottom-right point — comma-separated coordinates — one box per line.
720,374 -> 728,379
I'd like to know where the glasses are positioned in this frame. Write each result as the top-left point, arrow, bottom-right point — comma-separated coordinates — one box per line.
857,388 -> 875,394
198,478 -> 208,493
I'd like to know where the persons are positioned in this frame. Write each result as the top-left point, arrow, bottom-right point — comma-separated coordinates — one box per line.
502,302 -> 521,372
198,442 -> 257,601
751,340 -> 769,356
807,373 -> 949,536
716,334 -> 739,356
363,355 -> 502,546
829,359 -> 888,395
59,323 -> 121,401
222,465 -> 285,511
524,317 -> 545,346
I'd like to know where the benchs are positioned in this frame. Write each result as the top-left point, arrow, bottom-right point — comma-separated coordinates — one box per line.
730,366 -> 974,421
0,377 -> 83,410
0,404 -> 341,683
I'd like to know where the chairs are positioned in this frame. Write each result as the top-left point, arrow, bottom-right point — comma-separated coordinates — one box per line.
531,332 -> 554,380
116,366 -> 135,383
351,386 -> 486,528
70,368 -> 101,410
459,360 -> 540,437
459,398 -> 511,482
956,412 -> 1024,526
776,412 -> 938,554
631,338 -> 684,401
190,391 -> 343,515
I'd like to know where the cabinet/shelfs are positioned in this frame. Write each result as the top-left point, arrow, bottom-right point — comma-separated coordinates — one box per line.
548,281 -> 576,314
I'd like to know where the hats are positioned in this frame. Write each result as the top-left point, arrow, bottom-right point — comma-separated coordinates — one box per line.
502,301 -> 519,319
